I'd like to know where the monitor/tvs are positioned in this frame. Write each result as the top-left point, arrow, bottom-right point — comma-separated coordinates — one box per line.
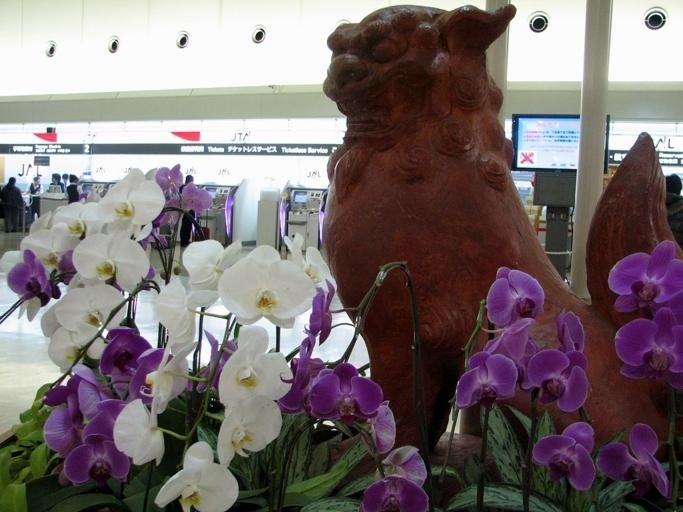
206,189 -> 216,198
294,192 -> 307,202
49,186 -> 61,193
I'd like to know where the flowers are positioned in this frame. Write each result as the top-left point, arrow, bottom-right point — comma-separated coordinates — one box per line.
6,157 -> 681,507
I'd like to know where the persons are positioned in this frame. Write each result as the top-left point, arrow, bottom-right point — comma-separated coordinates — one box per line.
66,174 -> 82,205
665,174 -> 682,250
50,172 -> 64,193
62,174 -> 69,192
0,177 -> 26,233
23,177 -> 44,223
179,175 -> 196,248
